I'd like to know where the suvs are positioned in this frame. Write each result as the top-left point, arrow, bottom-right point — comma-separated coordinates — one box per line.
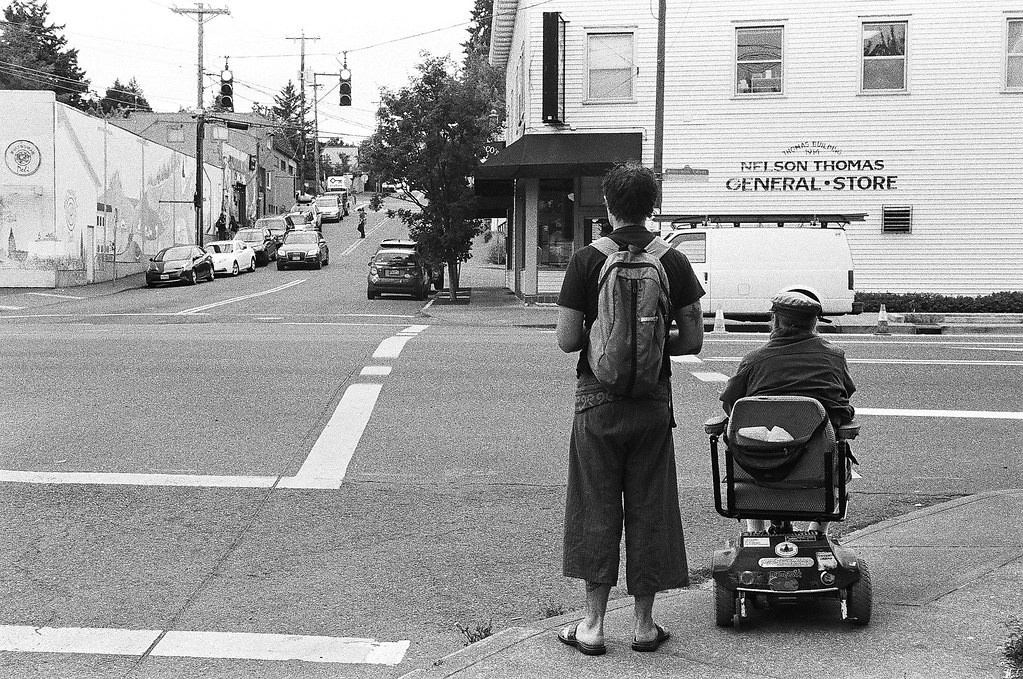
232,227 -> 278,267
254,213 -> 295,248
367,238 -> 433,301
284,189 -> 352,233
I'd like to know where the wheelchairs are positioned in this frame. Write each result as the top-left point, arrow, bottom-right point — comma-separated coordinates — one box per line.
705,396 -> 872,629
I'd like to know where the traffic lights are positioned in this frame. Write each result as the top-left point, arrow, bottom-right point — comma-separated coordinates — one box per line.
339,68 -> 352,106
220,69 -> 233,107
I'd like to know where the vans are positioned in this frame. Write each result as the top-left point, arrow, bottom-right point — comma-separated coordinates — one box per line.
652,210 -> 870,333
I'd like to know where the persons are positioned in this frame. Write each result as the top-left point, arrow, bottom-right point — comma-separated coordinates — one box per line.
356,208 -> 367,238
553,159 -> 706,654
718,285 -> 857,532
216,213 -> 228,241
228,216 -> 238,240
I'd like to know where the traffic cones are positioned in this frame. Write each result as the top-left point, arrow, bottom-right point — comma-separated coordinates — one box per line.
709,301 -> 729,333
873,303 -> 891,335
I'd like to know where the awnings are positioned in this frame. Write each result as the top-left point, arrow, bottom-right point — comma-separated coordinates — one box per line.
454,133 -> 642,219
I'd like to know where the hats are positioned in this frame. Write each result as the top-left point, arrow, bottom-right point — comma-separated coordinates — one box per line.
768,292 -> 821,320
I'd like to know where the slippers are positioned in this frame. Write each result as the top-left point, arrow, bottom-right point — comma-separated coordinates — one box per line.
559,624 -> 606,656
631,624 -> 670,652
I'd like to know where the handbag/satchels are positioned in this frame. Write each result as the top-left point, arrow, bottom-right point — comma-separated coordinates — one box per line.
729,414 -> 830,483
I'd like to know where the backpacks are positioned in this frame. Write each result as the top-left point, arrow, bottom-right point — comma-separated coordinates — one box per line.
586,235 -> 671,397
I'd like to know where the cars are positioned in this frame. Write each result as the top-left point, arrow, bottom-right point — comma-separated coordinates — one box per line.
202,239 -> 257,277
276,227 -> 330,270
146,243 -> 215,289
